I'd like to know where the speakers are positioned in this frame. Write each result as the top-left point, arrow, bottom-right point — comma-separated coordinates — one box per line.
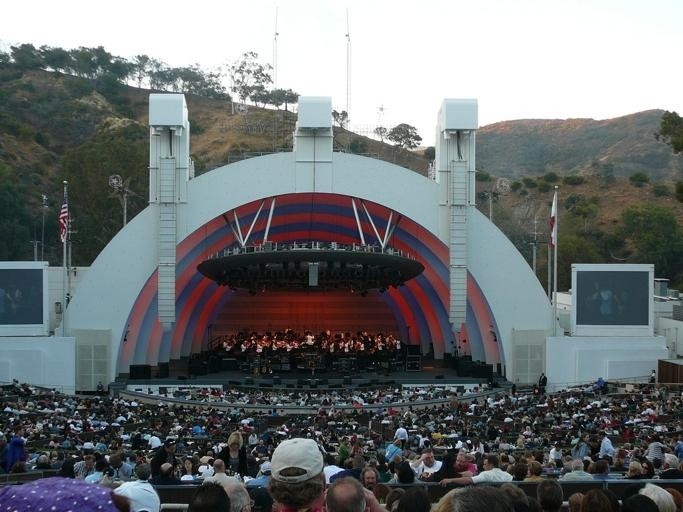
436,375 -> 444,378
228,377 -> 394,388
444,352 -> 493,379
497,363 -> 500,377
130,365 -> 151,378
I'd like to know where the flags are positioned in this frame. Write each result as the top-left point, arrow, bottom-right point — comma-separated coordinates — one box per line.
58,190 -> 70,242
548,192 -> 559,248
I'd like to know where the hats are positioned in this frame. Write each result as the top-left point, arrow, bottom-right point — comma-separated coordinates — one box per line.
82,442 -> 95,449
269,436 -> 325,485
